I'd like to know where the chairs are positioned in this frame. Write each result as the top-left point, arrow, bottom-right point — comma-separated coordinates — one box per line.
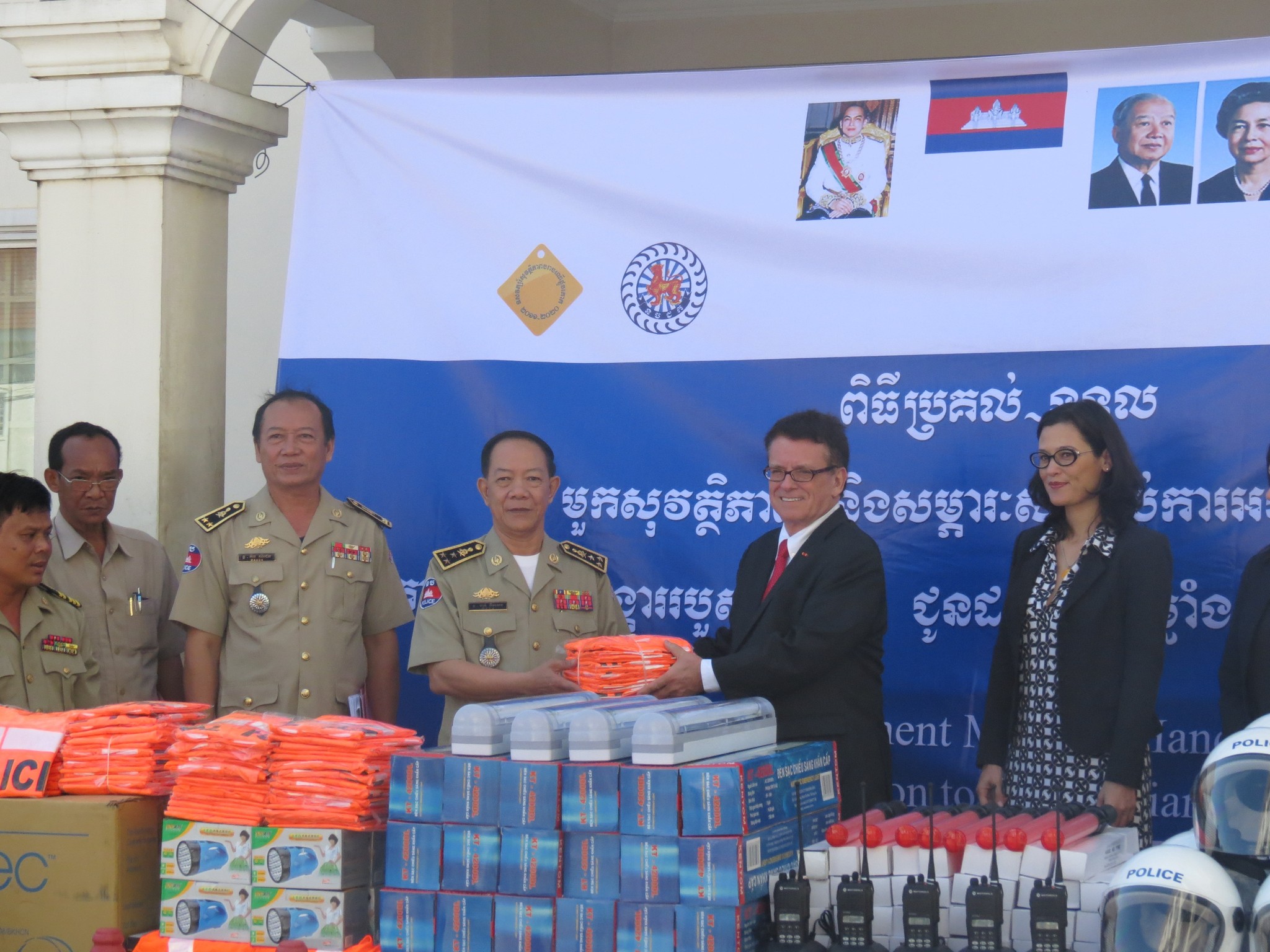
797,123 -> 895,218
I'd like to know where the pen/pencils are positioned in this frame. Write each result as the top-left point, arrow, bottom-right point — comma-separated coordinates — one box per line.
129,598 -> 132,616
137,587 -> 141,612
133,592 -> 137,611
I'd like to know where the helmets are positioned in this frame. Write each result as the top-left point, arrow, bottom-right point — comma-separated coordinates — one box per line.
1100,713 -> 1270,952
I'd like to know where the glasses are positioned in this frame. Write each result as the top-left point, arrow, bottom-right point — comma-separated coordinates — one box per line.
58,472 -> 121,492
763,465 -> 837,482
1030,448 -> 1097,469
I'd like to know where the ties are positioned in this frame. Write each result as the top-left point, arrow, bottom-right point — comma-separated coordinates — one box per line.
1140,174 -> 1156,206
762,540 -> 789,600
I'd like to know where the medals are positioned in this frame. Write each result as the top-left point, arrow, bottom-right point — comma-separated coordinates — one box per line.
249,593 -> 270,614
479,648 -> 500,668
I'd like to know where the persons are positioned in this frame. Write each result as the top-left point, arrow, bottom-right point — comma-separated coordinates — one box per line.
41,422 -> 186,710
1088,94 -> 1193,209
636,410 -> 895,820
975,398 -> 1173,851
407,431 -> 631,747
0,472 -> 101,714
1197,82 -> 1270,204
795,105 -> 887,221
1220,445 -> 1270,741
169,390 -> 416,725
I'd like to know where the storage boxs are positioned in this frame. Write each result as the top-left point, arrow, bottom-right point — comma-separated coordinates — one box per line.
0,793 -> 170,952
768,827 -> 1140,952
160,818 -> 386,952
379,741 -> 841,952
